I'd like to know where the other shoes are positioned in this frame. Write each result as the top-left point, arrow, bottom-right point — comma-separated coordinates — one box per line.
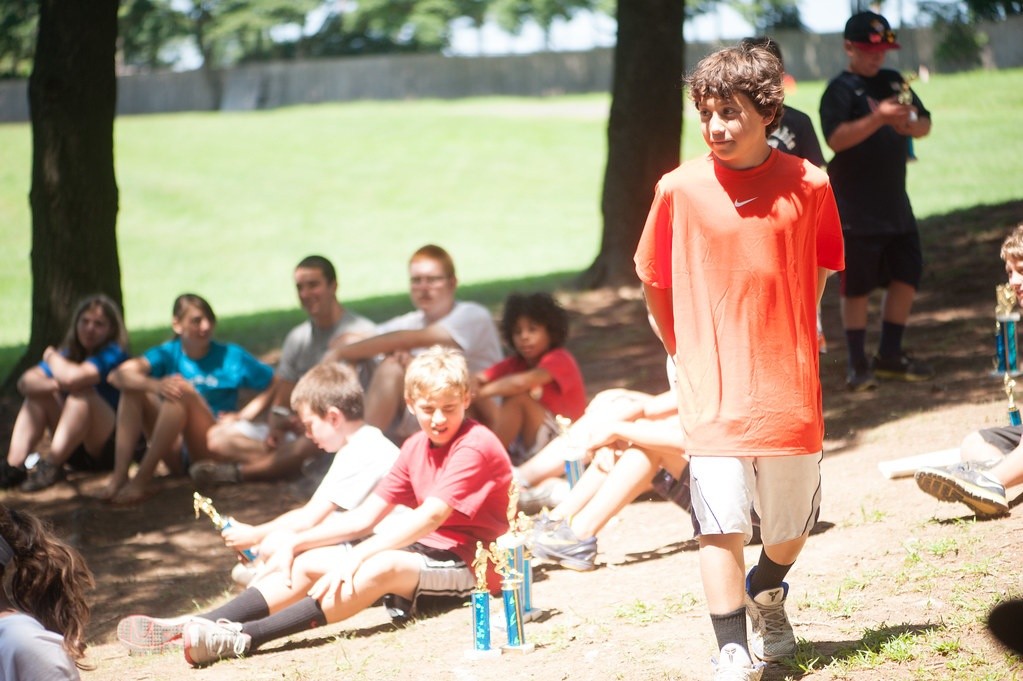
191,462 -> 238,485
18,460 -> 64,492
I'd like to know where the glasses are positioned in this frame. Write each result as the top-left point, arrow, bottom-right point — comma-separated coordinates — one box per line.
848,31 -> 899,45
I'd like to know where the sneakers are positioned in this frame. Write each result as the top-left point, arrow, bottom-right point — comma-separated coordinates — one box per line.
711,644 -> 766,681
916,466 -> 1010,518
874,352 -> 934,382
184,614 -> 251,667
844,362 -> 876,393
746,564 -> 795,661
116,611 -> 200,651
522,510 -> 598,571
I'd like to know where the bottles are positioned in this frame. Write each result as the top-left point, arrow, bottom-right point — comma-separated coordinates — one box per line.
898,84 -> 912,107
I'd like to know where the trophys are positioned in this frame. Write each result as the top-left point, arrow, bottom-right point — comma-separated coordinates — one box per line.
551,415 -> 585,493
470,481 -> 531,650
897,83 -> 917,162
998,374 -> 1022,429
193,491 -> 260,562
990,280 -> 1021,375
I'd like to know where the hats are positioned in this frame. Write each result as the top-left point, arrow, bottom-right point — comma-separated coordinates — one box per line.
843,11 -> 901,53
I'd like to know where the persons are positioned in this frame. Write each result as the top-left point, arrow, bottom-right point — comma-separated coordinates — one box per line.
504,288 -> 821,573
324,245 -> 506,452
0,296 -> 149,495
209,255 -> 387,481
116,346 -> 512,667
0,506 -> 96,681
741,38 -> 828,354
91,294 -> 278,506
632,45 -> 846,680
818,10 -> 937,393
462,291 -> 587,459
914,222 -> 1023,515
233,361 -> 402,586
